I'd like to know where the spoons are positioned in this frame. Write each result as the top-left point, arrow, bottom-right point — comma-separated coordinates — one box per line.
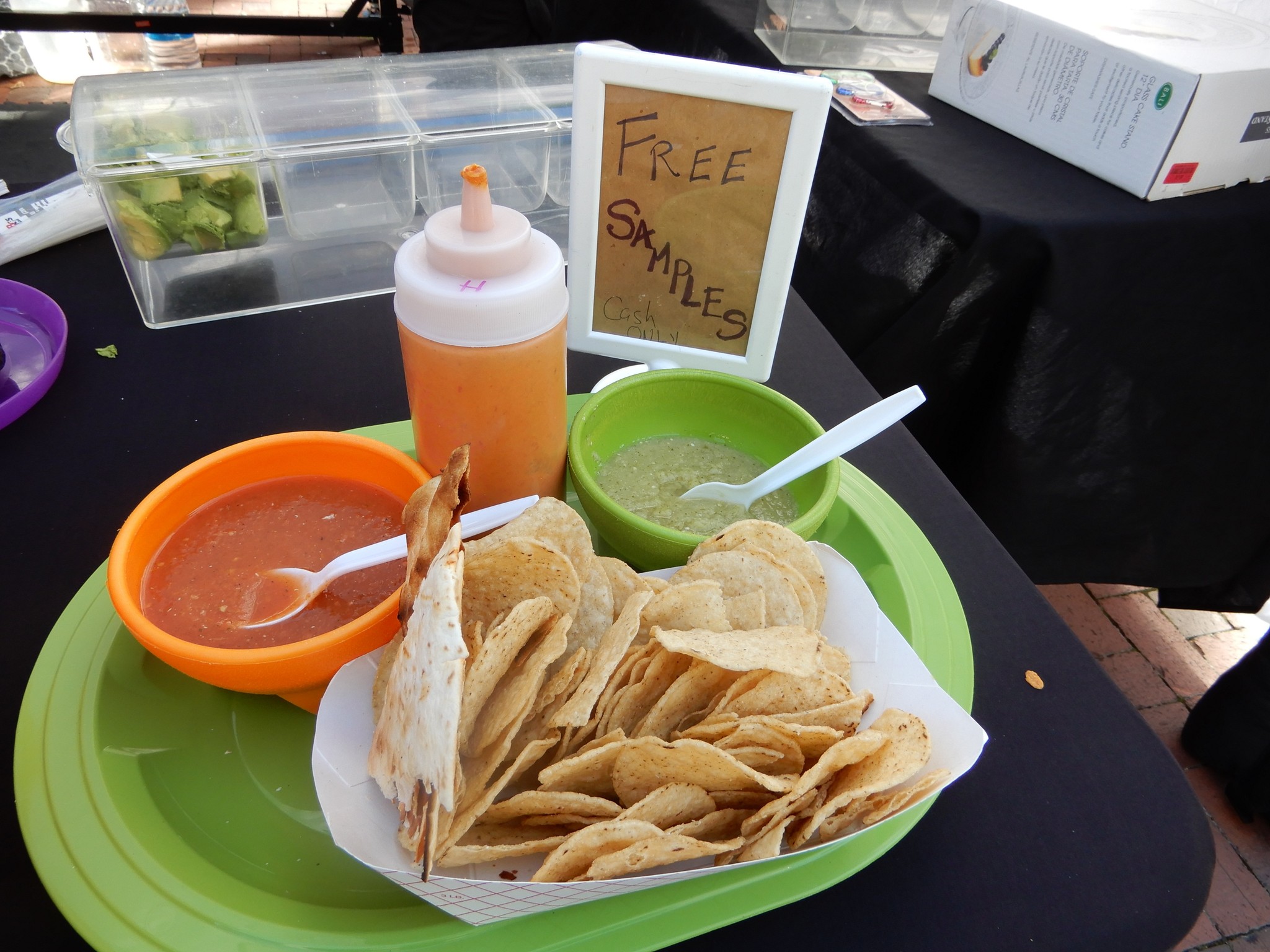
235,494 -> 542,631
679,384 -> 927,513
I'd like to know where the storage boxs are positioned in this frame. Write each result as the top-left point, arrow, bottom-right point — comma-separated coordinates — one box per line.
705,0 -> 949,73
69,38 -> 639,330
927,0 -> 1270,204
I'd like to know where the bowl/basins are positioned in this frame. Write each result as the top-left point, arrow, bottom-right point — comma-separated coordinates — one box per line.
106,430 -> 434,717
566,368 -> 840,571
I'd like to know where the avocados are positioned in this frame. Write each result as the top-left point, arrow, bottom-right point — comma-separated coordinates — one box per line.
100,116 -> 265,259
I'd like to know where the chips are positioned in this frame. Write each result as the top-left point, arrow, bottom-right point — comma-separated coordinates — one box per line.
375,440 -> 948,882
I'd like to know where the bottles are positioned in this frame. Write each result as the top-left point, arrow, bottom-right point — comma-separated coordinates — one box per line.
392,168 -> 570,541
136,0 -> 201,71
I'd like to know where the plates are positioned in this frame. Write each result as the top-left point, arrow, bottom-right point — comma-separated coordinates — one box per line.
0,278 -> 69,431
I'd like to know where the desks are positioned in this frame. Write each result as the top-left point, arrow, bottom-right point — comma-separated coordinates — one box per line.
384,0 -> 1270,614
1,94 -> 1218,950
